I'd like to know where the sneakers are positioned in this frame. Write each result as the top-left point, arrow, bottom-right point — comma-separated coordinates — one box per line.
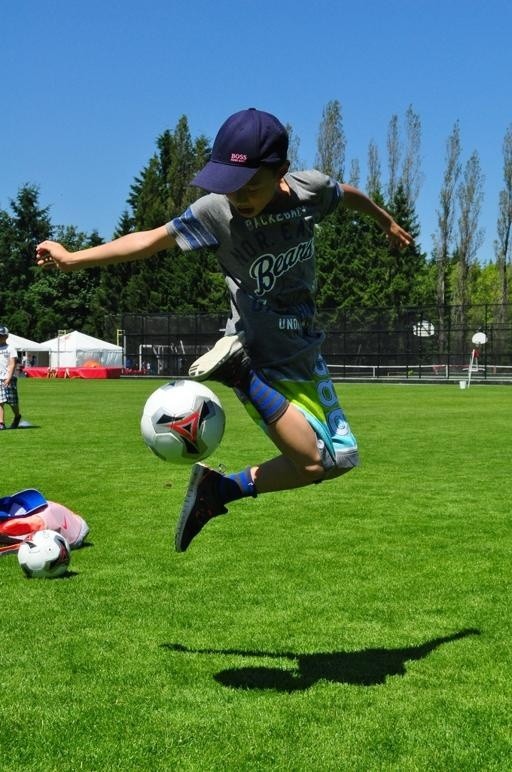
173,462 -> 232,553
0,414 -> 22,430
186,327 -> 256,390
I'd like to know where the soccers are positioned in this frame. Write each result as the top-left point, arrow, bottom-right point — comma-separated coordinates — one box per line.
18,528 -> 72,580
140,380 -> 226,464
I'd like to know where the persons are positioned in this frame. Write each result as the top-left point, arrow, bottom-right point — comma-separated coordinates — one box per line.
0,325 -> 22,429
35,112 -> 413,553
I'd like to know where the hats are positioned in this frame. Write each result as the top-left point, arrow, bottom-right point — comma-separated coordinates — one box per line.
188,107 -> 291,195
0,487 -> 50,521
0,325 -> 8,338
